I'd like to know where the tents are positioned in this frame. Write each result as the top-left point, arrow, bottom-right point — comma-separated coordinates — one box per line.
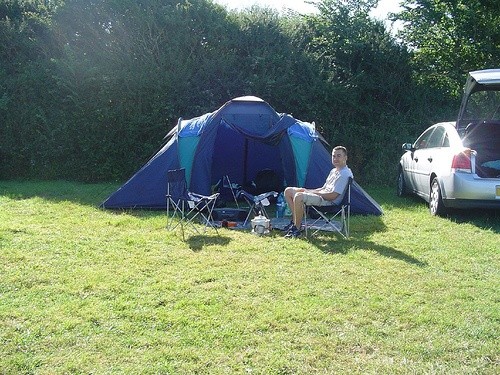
98,95 -> 384,218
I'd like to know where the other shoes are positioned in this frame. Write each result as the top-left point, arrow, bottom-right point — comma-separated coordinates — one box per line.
281,219 -> 302,239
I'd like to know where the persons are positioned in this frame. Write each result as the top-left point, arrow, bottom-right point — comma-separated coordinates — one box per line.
281,146 -> 353,238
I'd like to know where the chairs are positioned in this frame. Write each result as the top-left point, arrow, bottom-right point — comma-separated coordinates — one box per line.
166,167 -> 220,242
229,176 -> 280,225
304,175 -> 352,237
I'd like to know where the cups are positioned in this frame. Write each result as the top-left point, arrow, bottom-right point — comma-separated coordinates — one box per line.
256,225 -> 265,234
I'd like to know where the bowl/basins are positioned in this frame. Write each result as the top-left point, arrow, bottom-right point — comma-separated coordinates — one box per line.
251,211 -> 271,229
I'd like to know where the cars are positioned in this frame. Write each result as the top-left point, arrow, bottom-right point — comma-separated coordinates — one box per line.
397,69 -> 500,218
